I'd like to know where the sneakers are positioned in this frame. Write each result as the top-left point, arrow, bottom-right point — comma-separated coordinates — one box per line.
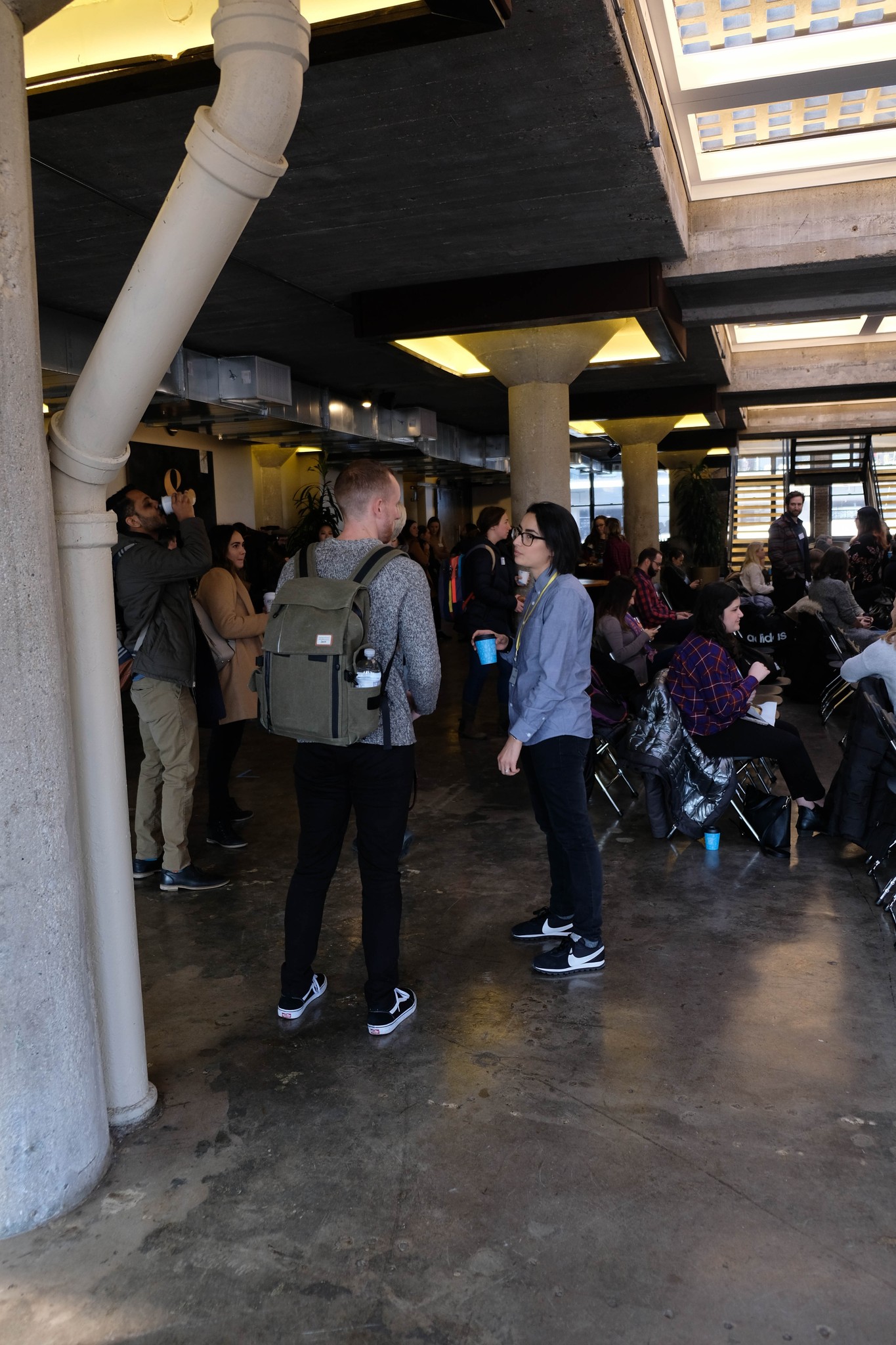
279,971 -> 328,1019
511,906 -> 576,940
367,987 -> 417,1035
531,933 -> 606,975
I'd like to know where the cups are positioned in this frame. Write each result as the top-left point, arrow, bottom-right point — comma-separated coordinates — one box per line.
768,568 -> 772,576
158,488 -> 184,517
262,592 -> 276,613
473,633 -> 499,665
888,551 -> 892,558
705,825 -> 722,850
519,570 -> 531,585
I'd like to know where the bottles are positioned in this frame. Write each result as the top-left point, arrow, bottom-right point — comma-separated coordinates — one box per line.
352,648 -> 382,688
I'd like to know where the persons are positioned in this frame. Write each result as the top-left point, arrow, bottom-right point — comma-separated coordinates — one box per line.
198,523 -> 270,847
106,485 -> 230,891
231,516 -> 479,641
274,461 -> 442,1034
471,501 -> 607,977
583,491 -> 896,835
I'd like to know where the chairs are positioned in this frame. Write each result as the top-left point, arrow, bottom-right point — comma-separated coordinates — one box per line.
593,569 -> 895,944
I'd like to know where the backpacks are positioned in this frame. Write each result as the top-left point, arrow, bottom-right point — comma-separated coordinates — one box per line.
447,544 -> 496,621
190,594 -> 239,673
586,665 -> 632,736
251,541 -> 416,746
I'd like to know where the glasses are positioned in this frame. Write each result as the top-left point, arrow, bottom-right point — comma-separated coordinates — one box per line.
650,559 -> 661,567
595,523 -> 605,528
511,526 -> 547,547
855,517 -> 860,522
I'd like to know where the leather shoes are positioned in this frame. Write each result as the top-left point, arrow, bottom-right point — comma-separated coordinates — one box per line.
796,802 -> 828,838
207,830 -> 248,848
228,810 -> 255,822
130,857 -> 165,878
159,867 -> 231,892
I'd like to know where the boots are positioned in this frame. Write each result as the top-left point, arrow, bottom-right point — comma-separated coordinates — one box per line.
496,701 -> 509,737
457,700 -> 487,739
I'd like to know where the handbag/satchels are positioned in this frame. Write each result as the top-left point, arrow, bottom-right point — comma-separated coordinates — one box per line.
740,781 -> 792,860
117,635 -> 135,691
